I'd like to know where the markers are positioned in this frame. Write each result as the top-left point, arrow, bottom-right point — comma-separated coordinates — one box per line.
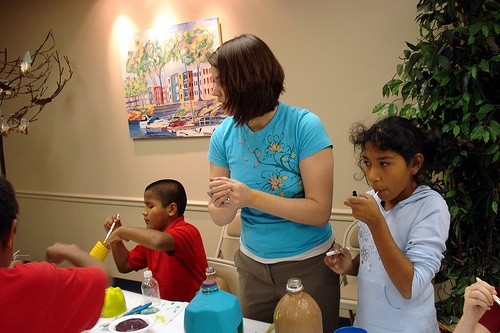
325,246 -> 350,257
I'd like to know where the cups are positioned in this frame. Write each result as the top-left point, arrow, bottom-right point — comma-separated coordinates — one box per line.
108,314 -> 154,333
333,326 -> 369,333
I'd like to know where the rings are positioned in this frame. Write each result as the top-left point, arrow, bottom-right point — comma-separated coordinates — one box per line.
207,188 -> 213,196
225,198 -> 231,203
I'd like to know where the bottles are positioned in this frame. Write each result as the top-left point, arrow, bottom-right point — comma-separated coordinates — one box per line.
274,278 -> 323,333
200,266 -> 232,297
141,270 -> 164,325
183,280 -> 245,333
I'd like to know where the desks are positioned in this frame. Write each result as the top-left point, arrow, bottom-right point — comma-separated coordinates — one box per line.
84,289 -> 270,333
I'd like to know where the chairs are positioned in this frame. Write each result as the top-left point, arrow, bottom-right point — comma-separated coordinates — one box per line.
339,219 -> 358,326
207,209 -> 240,296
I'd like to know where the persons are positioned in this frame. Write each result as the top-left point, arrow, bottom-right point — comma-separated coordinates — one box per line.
206,34 -> 343,333
0,175 -> 107,333
323,117 -> 500,333
101,179 -> 210,303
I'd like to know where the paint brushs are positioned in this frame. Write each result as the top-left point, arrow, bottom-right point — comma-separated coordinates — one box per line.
103,213 -> 120,245
475,277 -> 500,306
